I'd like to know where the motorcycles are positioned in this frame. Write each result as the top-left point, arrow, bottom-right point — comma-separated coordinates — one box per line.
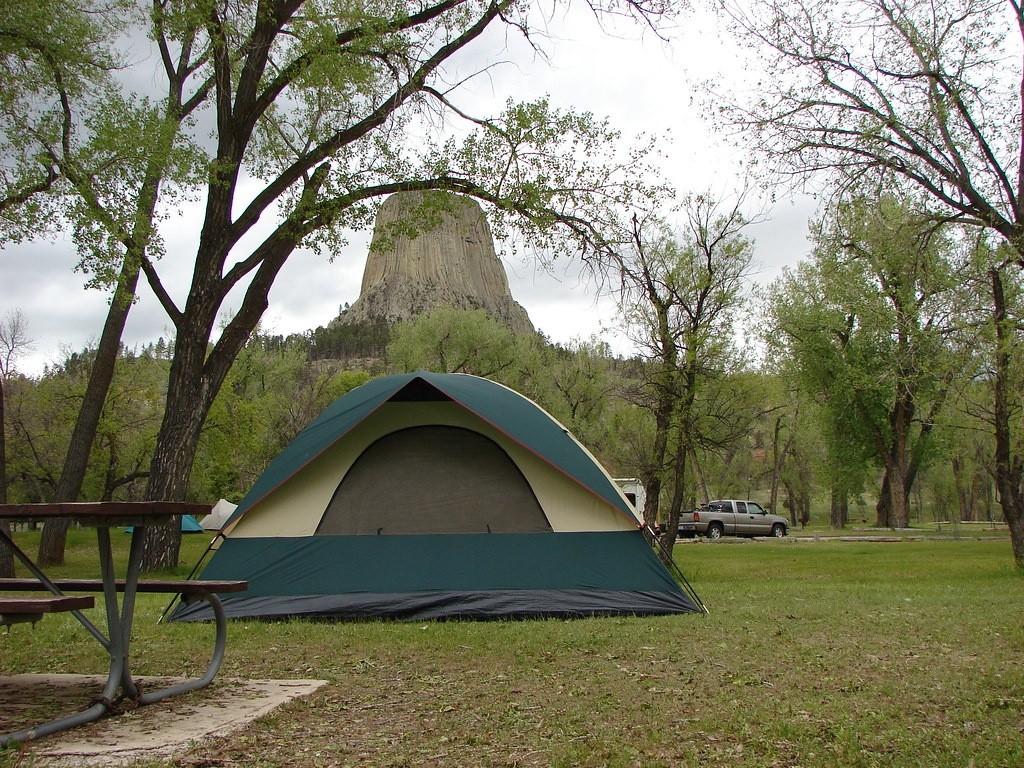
694,504 -> 725,512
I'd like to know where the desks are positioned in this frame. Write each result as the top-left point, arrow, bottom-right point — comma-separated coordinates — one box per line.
0,502 -> 214,711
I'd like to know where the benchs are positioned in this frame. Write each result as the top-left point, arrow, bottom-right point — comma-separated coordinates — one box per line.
0,594 -> 125,749
0,577 -> 250,704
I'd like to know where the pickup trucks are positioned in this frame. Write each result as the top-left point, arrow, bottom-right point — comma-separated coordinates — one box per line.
669,499 -> 790,539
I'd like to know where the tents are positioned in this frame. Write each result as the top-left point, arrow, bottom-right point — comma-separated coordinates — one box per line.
198,498 -> 239,531
155,373 -> 709,630
125,513 -> 206,533
